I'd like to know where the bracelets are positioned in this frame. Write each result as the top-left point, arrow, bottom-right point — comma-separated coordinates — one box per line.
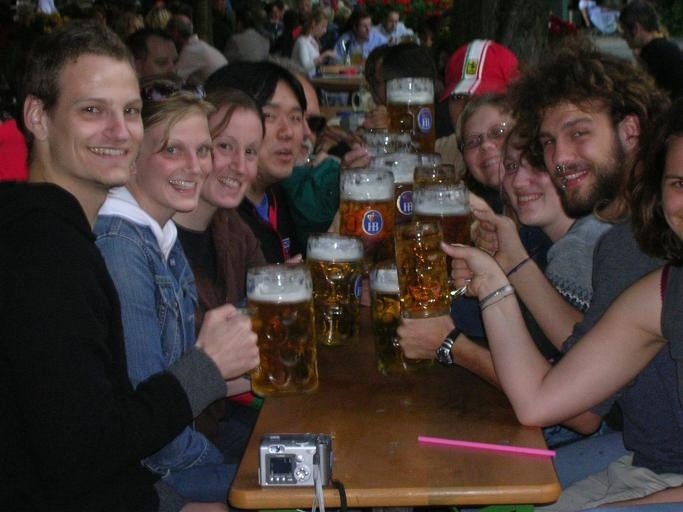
507,257 -> 529,279
479,286 -> 514,311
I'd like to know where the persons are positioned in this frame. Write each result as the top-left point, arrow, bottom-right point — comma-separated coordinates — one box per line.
89,76 -> 258,498
2,1 -> 682,206
396,39 -> 682,512
474,129 -> 651,488
182,85 -> 266,365
1,20 -> 227,511
441,102 -> 681,512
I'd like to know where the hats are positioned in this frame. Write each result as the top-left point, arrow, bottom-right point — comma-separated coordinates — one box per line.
439,40 -> 524,103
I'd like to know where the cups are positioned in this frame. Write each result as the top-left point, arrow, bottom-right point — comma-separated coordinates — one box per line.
369,261 -> 436,374
337,79 -> 472,275
248,258 -> 321,396
307,232 -> 364,346
394,218 -> 473,319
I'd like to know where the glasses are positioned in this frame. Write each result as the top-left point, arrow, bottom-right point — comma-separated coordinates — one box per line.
461,122 -> 514,150
142,81 -> 207,102
302,114 -> 329,134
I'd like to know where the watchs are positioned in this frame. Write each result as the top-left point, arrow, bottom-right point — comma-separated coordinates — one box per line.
438,329 -> 462,366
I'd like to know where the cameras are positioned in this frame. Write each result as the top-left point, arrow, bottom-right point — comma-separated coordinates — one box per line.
258,432 -> 333,487
